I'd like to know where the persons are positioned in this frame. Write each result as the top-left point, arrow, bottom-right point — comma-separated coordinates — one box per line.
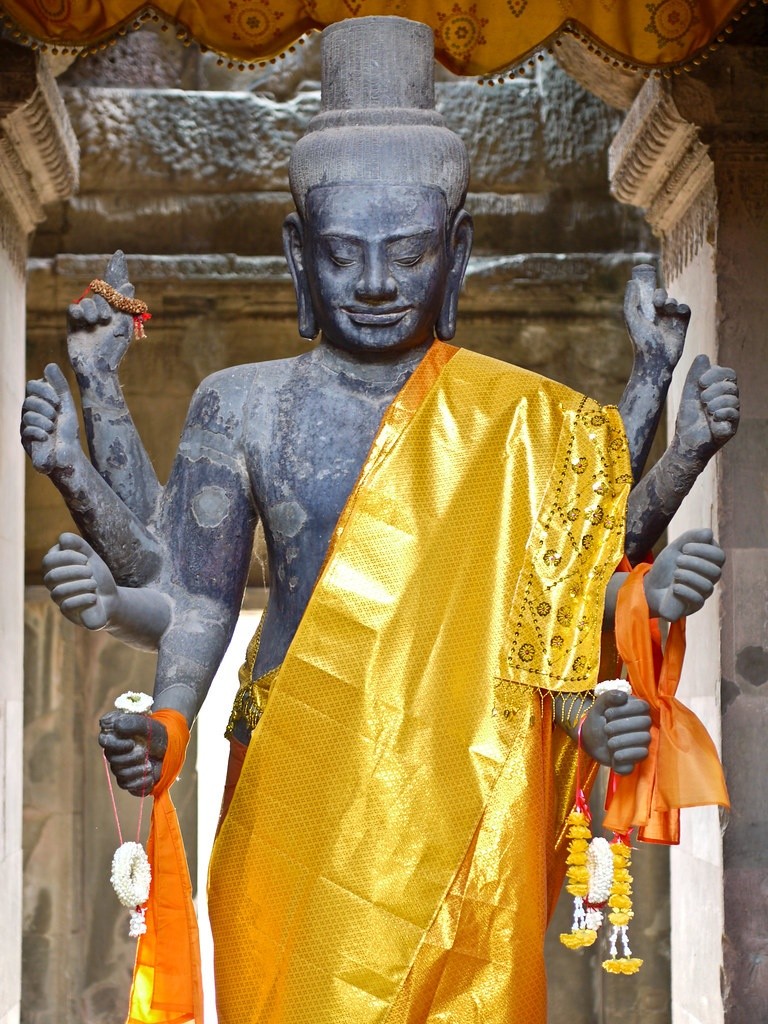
21,110 -> 742,1024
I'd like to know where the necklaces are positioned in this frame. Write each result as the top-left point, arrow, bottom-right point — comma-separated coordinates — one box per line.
559,709 -> 643,975
101,711 -> 153,939
